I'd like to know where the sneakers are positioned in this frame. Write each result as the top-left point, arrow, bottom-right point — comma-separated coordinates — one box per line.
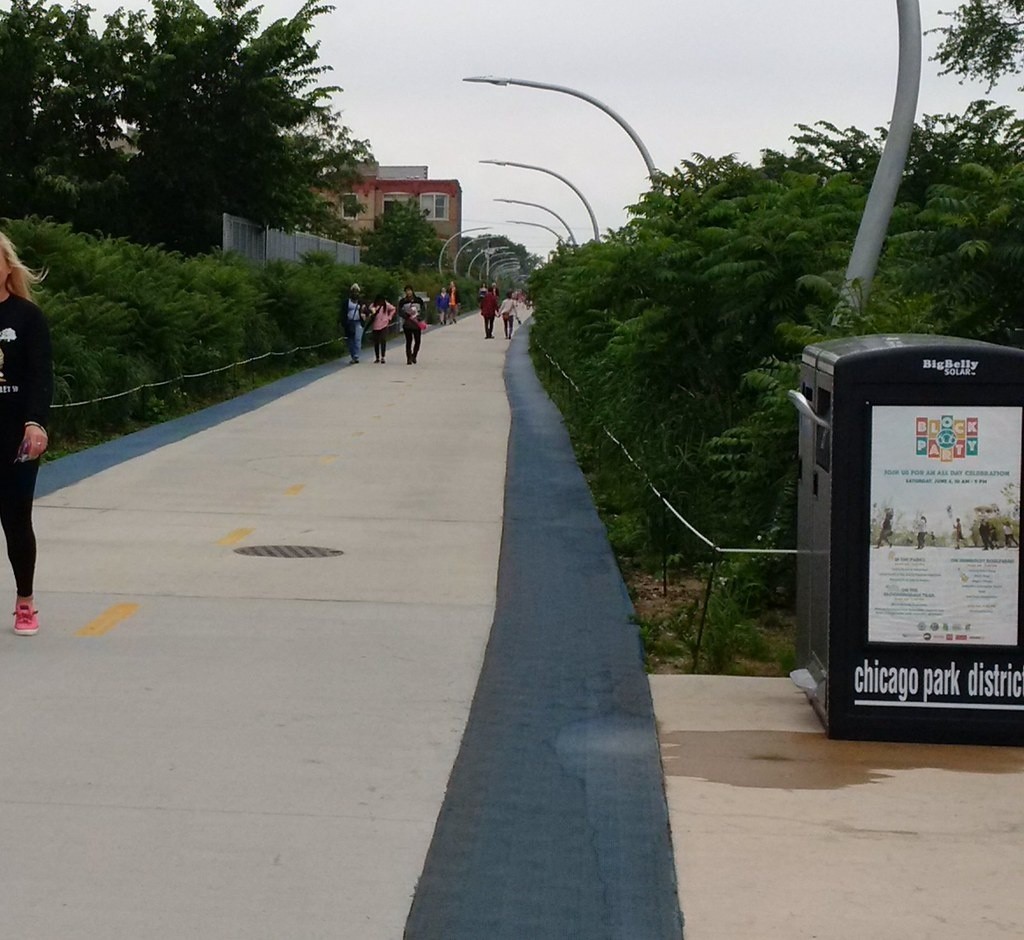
13,603 -> 40,635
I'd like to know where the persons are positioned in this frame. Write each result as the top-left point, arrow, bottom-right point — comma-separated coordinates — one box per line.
342,278 -> 533,366
875,508 -> 1019,549
0,235 -> 54,637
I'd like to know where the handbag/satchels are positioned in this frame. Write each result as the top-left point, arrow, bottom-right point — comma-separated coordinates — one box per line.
503,312 -> 510,321
344,320 -> 354,337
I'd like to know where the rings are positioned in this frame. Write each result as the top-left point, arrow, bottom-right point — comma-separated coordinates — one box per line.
37,442 -> 42,445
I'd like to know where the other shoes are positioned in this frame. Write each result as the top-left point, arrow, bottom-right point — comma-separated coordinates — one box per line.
381,359 -> 385,363
412,355 -> 416,363
374,359 -> 379,363
408,358 -> 411,364
350,359 -> 359,363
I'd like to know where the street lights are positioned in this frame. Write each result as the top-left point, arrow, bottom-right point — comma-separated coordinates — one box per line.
454,233 -> 500,275
467,245 -> 524,285
476,158 -> 601,245
437,226 -> 493,279
459,74 -> 661,194
493,196 -> 581,249
505,220 -> 567,249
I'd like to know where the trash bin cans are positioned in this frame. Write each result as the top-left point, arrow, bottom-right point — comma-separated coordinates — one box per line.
786,333 -> 1024,747
